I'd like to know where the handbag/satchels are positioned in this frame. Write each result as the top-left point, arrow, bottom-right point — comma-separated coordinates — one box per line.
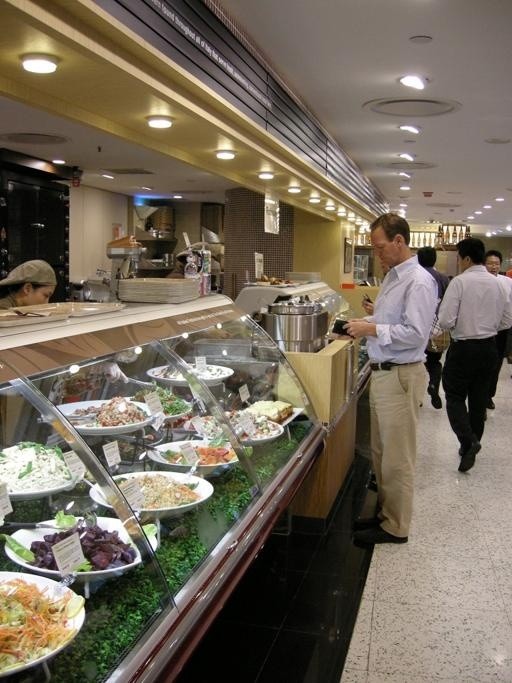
428,328 -> 450,353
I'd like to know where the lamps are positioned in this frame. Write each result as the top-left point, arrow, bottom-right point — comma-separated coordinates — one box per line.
20,53 -> 59,74
146,115 -> 174,129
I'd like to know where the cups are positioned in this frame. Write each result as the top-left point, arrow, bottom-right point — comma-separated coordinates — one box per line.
163,253 -> 176,268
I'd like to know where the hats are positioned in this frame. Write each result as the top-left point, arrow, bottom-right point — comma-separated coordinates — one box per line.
0,260 -> 57,286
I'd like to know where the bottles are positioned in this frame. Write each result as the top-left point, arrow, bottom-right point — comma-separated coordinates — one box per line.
360,235 -> 369,245
412,225 -> 470,248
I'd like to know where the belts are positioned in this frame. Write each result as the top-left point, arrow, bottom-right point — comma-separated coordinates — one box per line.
371,362 -> 422,370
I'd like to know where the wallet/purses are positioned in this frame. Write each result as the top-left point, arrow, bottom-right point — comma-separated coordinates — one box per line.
332,320 -> 356,339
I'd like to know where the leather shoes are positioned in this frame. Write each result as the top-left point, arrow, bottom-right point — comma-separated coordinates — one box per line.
487,399 -> 495,409
355,516 -> 407,542
427,384 -> 442,409
458,440 -> 481,471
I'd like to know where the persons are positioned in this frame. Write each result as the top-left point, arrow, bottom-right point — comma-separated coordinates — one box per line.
417,246 -> 451,409
484,250 -> 512,423
342,212 -> 438,544
1,260 -> 58,309
438,238 -> 507,472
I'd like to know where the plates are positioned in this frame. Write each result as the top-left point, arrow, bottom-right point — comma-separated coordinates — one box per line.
244,407 -> 305,426
146,363 -> 234,388
1,570 -> 86,677
89,471 -> 215,521
56,400 -> 153,437
5,475 -> 77,498
4,516 -> 157,582
147,441 -> 241,477
184,414 -> 284,446
